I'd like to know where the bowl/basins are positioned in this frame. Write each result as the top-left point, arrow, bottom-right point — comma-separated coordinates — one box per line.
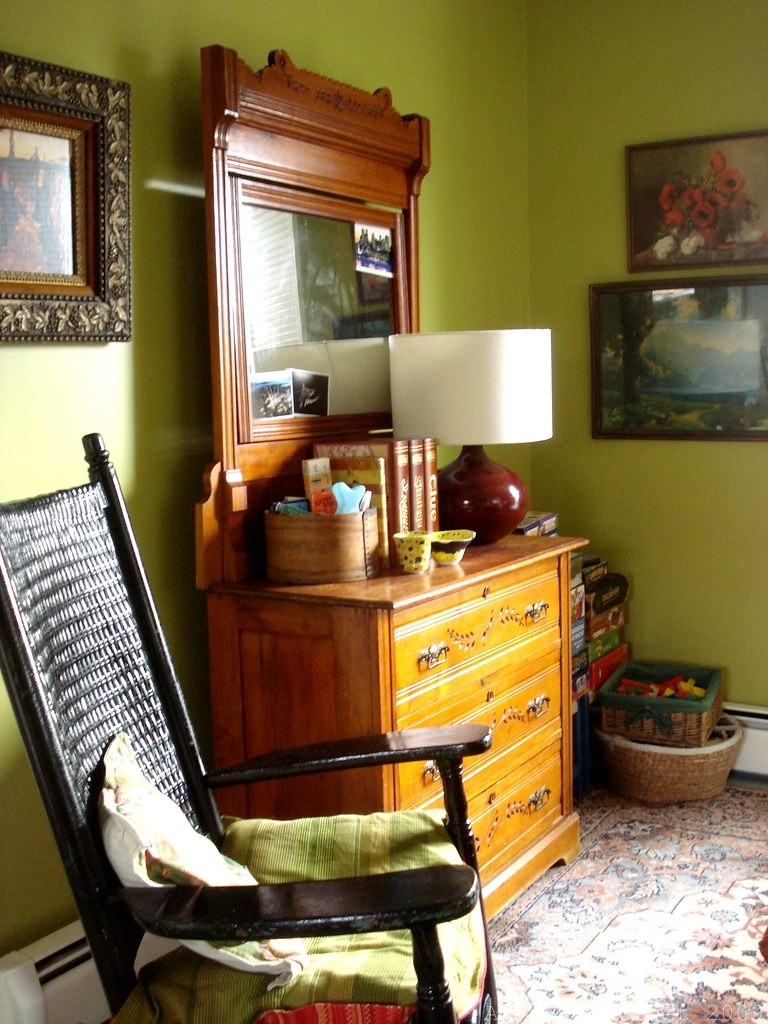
430,530 -> 477,566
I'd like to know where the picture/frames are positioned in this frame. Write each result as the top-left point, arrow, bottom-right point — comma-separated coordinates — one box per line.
349,224 -> 390,304
331,308 -> 390,338
0,50 -> 132,343
624,128 -> 768,272
589,273 -> 768,442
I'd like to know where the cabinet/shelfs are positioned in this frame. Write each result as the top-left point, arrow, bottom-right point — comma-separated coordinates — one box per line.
203,533 -> 591,915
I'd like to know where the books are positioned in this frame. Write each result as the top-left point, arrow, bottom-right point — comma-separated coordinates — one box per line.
312,438 -> 439,568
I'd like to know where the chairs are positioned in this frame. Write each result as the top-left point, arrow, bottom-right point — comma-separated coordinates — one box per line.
0,435 -> 500,1024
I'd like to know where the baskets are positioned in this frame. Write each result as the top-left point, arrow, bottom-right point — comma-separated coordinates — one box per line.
595,712 -> 743,807
597,660 -> 724,747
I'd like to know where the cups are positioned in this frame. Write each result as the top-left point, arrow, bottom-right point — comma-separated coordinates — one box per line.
393,531 -> 435,574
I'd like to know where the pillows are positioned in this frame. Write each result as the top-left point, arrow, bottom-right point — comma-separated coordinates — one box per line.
96,733 -> 312,991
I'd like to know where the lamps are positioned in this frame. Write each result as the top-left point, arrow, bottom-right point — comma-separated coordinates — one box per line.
253,337 -> 390,415
388,328 -> 555,546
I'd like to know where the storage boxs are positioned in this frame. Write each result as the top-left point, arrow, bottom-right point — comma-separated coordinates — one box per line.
515,509 -> 631,808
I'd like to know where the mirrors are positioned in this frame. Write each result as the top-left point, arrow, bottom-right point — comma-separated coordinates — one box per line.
199,44 -> 432,596
